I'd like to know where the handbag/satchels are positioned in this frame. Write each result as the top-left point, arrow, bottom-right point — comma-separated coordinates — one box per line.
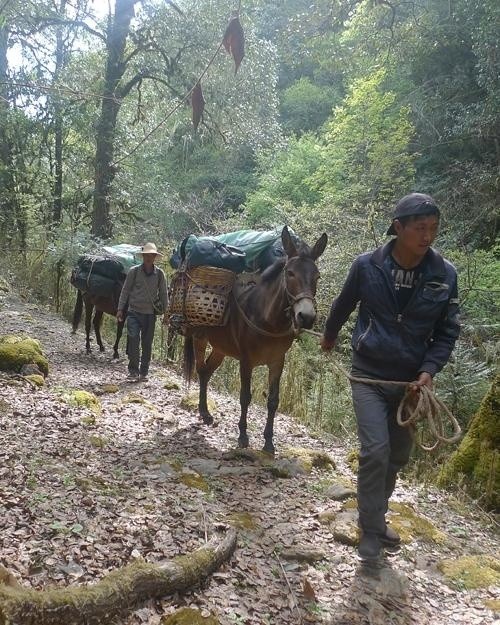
154,299 -> 163,315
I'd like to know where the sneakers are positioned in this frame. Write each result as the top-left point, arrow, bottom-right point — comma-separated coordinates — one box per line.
127,372 -> 139,383
358,534 -> 382,560
140,374 -> 149,382
357,520 -> 401,544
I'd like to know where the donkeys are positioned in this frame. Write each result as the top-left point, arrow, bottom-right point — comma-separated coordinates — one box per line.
179,225 -> 329,457
71,270 -> 165,361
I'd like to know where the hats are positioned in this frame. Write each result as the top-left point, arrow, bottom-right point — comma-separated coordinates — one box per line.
136,242 -> 165,260
386,193 -> 441,236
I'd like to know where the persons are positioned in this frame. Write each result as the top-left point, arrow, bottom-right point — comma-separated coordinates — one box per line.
319,189 -> 460,559
116,241 -> 171,382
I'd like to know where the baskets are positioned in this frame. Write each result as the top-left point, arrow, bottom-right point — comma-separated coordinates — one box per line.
163,265 -> 240,329
